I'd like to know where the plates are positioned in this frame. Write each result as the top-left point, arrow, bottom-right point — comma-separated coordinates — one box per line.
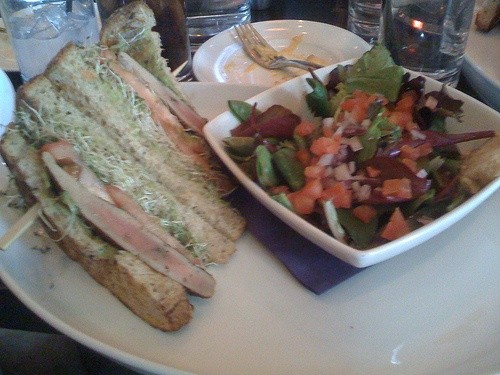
458,55 -> 500,115
1,82 -> 500,374
0,67 -> 17,137
461,0 -> 500,89
192,19 -> 373,80
201,57 -> 499,269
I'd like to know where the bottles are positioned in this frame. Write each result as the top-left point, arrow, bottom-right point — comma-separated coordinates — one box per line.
95,0 -> 198,82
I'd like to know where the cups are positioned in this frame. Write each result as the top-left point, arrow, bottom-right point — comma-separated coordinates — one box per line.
184,0 -> 252,53
380,0 -> 476,89
347,0 -> 383,46
0,0 -> 101,85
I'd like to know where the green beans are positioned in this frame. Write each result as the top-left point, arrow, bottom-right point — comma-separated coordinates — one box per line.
225,98 -> 303,194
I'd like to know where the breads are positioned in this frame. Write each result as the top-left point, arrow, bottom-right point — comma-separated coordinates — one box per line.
0,0 -> 245,333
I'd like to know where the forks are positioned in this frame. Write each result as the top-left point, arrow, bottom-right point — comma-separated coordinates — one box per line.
233,21 -> 325,70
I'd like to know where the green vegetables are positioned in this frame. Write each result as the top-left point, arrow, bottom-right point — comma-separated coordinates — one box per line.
304,46 -> 467,247
